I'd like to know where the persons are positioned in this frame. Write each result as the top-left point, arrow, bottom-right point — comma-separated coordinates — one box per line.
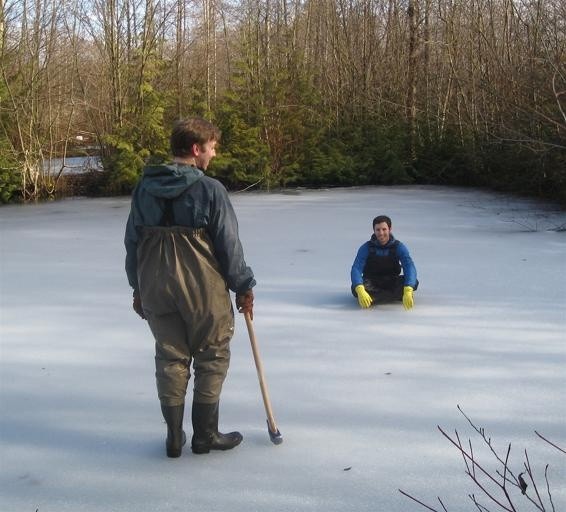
121,115 -> 257,458
349,214 -> 420,312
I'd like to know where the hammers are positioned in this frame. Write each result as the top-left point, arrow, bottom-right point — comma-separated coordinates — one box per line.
237,313 -> 283,445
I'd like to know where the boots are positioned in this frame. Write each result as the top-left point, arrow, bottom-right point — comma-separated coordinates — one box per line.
160,405 -> 187,457
191,402 -> 243,454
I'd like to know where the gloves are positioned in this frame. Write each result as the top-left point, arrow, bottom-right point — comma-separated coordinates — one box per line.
235,290 -> 254,320
355,285 -> 373,309
402,286 -> 414,310
132,297 -> 146,321
